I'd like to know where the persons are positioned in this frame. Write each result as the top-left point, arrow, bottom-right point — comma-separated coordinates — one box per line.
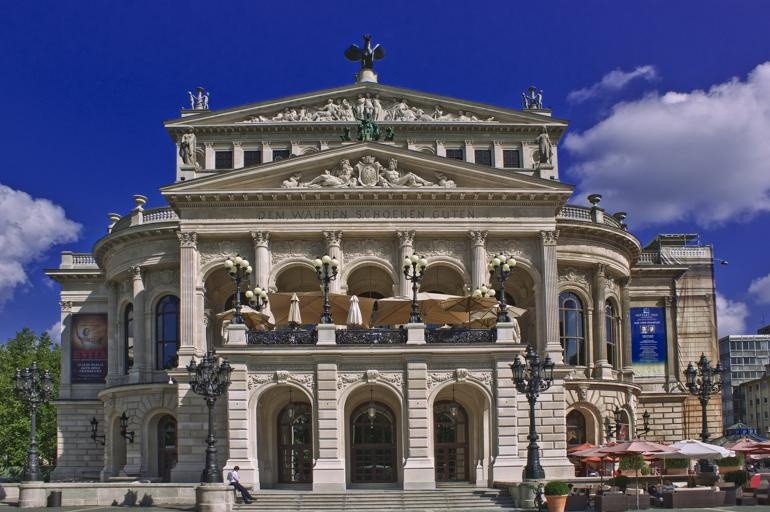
534,124 -> 554,167
534,88 -> 543,109
202,92 -> 210,109
520,92 -> 533,110
188,92 -> 197,112
230,466 -> 257,504
235,91 -> 495,189
181,126 -> 198,166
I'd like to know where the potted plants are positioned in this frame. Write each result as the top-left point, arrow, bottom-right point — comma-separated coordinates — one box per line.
619,454 -> 644,476
544,480 -> 570,512
665,458 -> 690,475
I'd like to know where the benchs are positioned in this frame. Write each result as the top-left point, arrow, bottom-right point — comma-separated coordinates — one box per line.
566,490 -> 718,512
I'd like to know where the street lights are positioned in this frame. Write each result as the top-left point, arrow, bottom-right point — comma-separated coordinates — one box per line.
225,255 -> 253,324
511,343 -> 555,479
683,352 -> 727,442
186,347 -> 235,483
11,361 -> 54,480
313,255 -> 340,324
473,283 -> 496,300
246,287 -> 269,312
404,252 -> 428,323
487,252 -> 517,321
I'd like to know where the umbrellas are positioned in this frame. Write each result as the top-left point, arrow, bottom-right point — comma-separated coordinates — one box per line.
566,420 -> 770,511
